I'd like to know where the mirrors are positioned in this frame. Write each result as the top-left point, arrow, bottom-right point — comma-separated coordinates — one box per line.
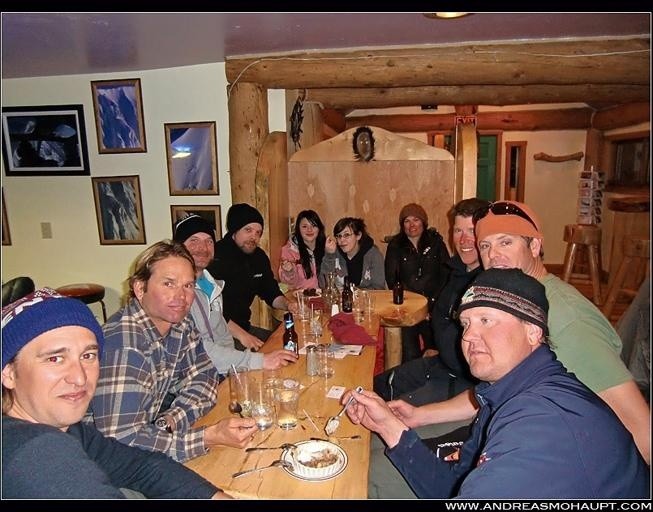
164,120 -> 220,196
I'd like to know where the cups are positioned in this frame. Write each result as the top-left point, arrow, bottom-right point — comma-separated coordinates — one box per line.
229,367 -> 249,413
310,309 -> 324,338
262,369 -> 284,383
297,292 -> 310,322
284,350 -> 298,387
251,383 -> 275,429
277,389 -> 299,428
236,376 -> 257,417
358,288 -> 370,310
364,295 -> 375,322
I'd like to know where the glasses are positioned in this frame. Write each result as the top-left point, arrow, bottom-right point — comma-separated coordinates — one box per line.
336,233 -> 359,238
472,202 -> 538,228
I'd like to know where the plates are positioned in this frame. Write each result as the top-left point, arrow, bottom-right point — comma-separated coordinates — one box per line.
280,438 -> 348,484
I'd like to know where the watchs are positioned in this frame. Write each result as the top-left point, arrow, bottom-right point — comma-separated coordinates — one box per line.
152,418 -> 172,432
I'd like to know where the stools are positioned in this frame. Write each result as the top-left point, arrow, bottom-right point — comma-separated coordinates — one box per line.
603,235 -> 650,321
560,224 -> 605,306
56,283 -> 107,324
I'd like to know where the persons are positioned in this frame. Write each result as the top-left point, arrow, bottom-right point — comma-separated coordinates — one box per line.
372,197 -> 491,408
340,268 -> 647,499
278,209 -> 325,293
1,286 -> 235,499
205,204 -> 302,351
171,214 -> 298,371
322,218 -> 385,290
369,200 -> 653,499
385,202 -> 448,300
79,239 -> 256,464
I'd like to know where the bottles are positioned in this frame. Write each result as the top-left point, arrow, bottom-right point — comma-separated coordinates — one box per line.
393,270 -> 404,305
342,277 -> 353,313
284,312 -> 299,361
322,272 -> 340,311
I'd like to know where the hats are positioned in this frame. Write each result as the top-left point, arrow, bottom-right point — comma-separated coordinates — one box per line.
226,203 -> 264,235
458,268 -> 549,331
174,214 -> 215,244
399,203 -> 428,226
475,200 -> 542,246
2,287 -> 104,370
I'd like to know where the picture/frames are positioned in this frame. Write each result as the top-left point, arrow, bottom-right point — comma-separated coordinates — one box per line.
2,186 -> 12,245
2,104 -> 91,176
90,78 -> 147,155
170,205 -> 222,242
91,175 -> 147,246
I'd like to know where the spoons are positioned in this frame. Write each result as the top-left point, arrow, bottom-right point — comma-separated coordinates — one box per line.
231,459 -> 292,479
228,401 -> 253,430
388,371 -> 397,400
246,442 -> 298,454
324,385 -> 364,437
